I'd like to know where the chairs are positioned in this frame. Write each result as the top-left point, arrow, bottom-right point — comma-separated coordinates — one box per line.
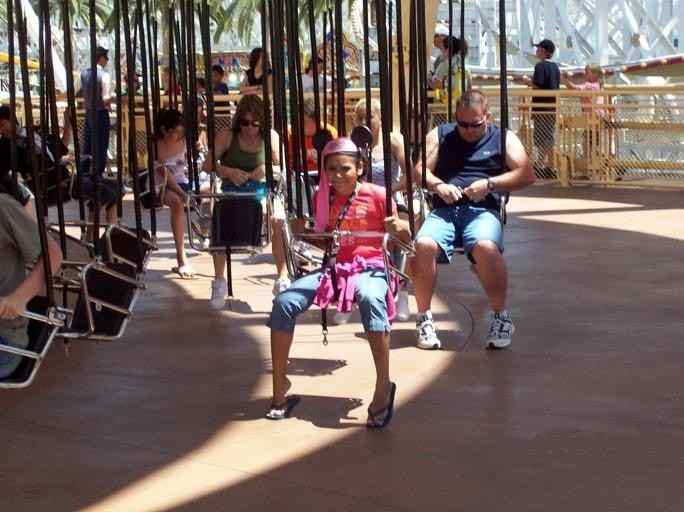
0,294 -> 66,390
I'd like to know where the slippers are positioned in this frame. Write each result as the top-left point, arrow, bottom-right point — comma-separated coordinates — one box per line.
172,264 -> 196,279
265,394 -> 300,419
366,383 -> 396,429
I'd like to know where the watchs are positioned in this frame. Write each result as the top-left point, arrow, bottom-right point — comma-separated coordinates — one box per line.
485,177 -> 497,194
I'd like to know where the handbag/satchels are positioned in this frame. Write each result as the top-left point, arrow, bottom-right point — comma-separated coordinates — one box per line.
213,200 -> 262,246
220,178 -> 268,200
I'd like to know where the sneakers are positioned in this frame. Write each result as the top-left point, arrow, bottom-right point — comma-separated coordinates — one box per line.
486,311 -> 516,351
615,168 -> 626,181
396,297 -> 410,321
415,310 -> 442,350
273,278 -> 291,296
574,175 -> 591,181
209,280 -> 226,310
532,162 -> 558,179
201,238 -> 210,249
333,306 -> 358,324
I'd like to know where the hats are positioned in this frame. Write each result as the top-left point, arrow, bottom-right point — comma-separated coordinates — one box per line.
124,73 -> 141,82
96,47 -> 108,58
312,125 -> 373,158
434,22 -> 449,35
532,39 -> 555,53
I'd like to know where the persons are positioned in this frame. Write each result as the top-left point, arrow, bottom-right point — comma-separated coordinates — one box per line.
266,135 -> 412,427
412,88 -> 536,350
427,22 -> 450,103
144,107 -> 221,279
0,171 -> 36,221
560,61 -> 628,180
285,97 -> 333,230
523,38 -> 560,181
331,97 -> 411,324
0,191 -> 63,381
116,45 -> 362,122
429,34 -> 472,128
0,105 -> 121,244
48,47 -> 116,180
201,93 -> 293,311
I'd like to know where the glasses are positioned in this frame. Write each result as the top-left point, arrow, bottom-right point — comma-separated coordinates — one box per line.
240,119 -> 262,127
456,113 -> 486,128
